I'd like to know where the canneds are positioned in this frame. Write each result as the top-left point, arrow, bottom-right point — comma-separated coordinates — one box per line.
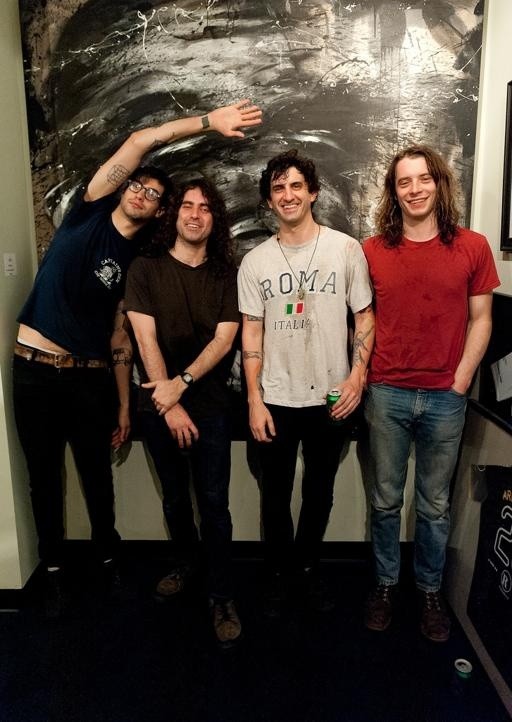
327,388 -> 342,426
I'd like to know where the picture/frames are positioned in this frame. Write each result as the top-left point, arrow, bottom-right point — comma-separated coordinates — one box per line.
500,80 -> 512,253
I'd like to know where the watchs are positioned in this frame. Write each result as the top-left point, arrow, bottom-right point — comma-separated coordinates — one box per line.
179,371 -> 194,386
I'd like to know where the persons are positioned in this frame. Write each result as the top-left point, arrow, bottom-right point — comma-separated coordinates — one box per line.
123,178 -> 246,648
360,147 -> 505,642
10,97 -> 263,616
235,150 -> 377,620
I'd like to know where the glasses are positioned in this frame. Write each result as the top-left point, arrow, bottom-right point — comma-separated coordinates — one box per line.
128,177 -> 162,201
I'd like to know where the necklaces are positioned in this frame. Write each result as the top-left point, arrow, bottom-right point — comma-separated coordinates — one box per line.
278,223 -> 320,300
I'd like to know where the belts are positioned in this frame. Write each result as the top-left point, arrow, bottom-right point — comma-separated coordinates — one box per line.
14,341 -> 107,368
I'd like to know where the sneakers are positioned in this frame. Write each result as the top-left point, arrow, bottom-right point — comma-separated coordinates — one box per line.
102,558 -> 140,613
295,564 -> 337,610
44,564 -> 69,620
209,594 -> 242,646
363,583 -> 393,631
420,591 -> 451,642
157,564 -> 194,597
260,565 -> 287,619
